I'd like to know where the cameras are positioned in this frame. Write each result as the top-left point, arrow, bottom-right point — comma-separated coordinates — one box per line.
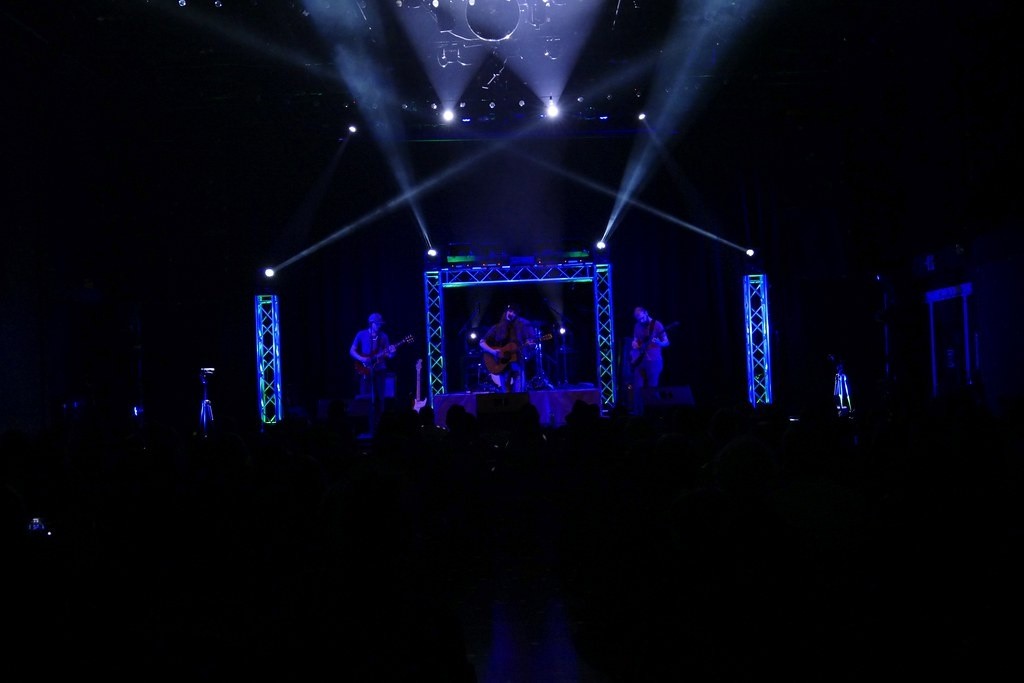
197,367 -> 215,377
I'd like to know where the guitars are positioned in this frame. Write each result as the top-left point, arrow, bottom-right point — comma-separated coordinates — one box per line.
353,333 -> 416,376
630,321 -> 682,366
483,332 -> 553,376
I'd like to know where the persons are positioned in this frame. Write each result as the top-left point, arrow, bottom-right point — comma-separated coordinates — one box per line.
0,400 -> 1024,683
480,302 -> 530,392
632,307 -> 669,414
350,313 -> 396,405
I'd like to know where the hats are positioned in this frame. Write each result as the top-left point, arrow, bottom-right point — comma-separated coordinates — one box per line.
504,303 -> 520,313
369,314 -> 384,323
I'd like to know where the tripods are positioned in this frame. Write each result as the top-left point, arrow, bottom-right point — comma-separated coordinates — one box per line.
523,328 -> 555,390
196,378 -> 217,435
